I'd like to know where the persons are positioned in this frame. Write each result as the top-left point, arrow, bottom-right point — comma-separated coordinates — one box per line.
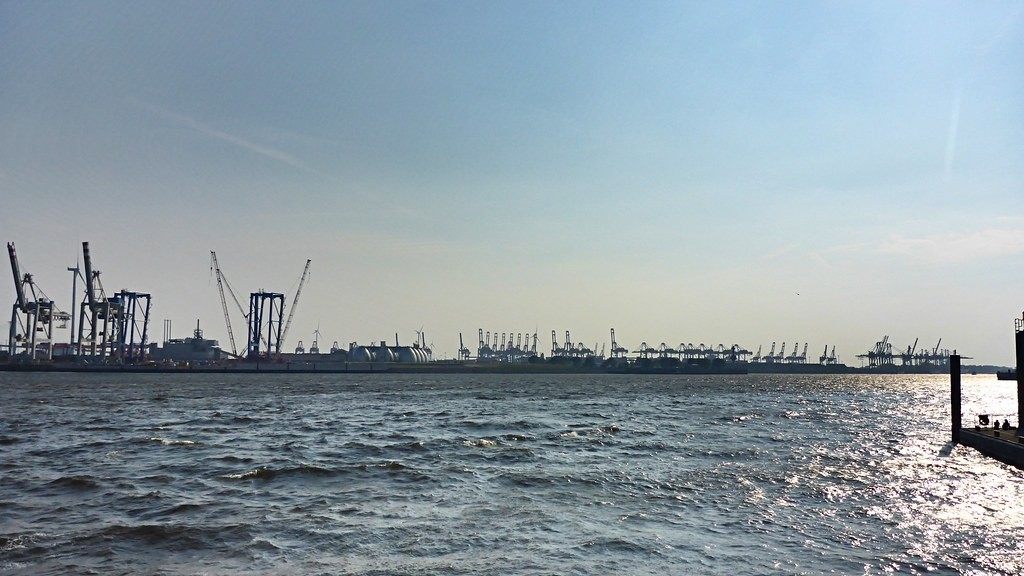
994,420 -> 1000,429
1002,419 -> 1010,431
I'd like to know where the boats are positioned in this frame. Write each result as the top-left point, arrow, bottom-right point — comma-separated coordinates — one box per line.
996,368 -> 1017,381
